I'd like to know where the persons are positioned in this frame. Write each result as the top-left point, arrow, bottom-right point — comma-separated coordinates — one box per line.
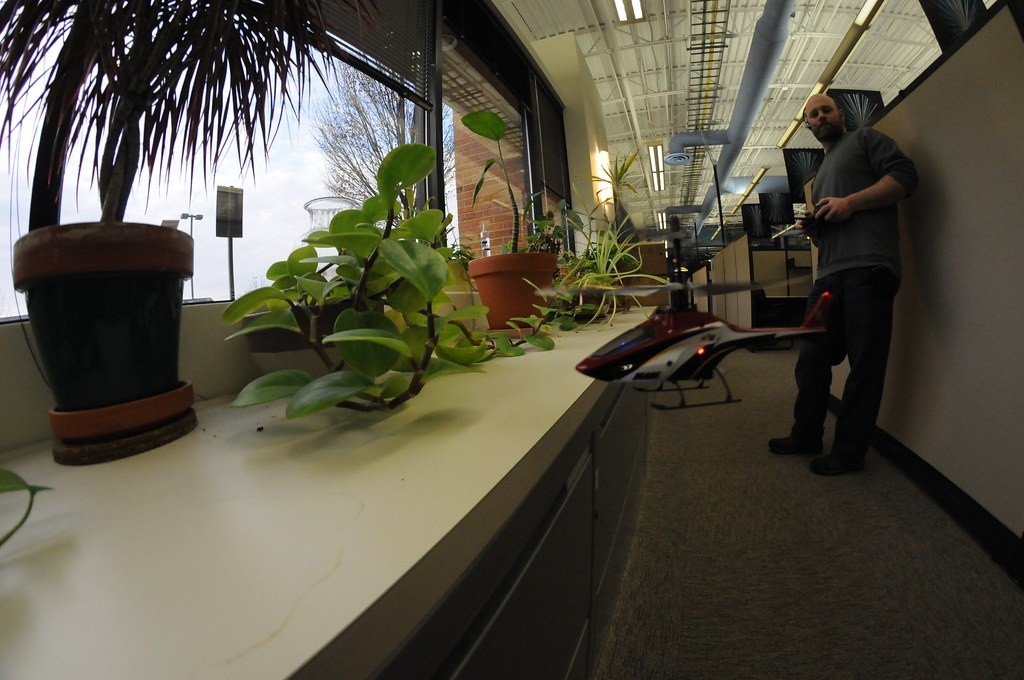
768,94 -> 920,476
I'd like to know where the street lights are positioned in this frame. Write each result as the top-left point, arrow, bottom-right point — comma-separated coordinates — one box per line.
181,213 -> 203,298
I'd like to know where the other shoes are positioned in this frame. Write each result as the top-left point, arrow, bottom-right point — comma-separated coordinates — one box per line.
811,450 -> 860,477
769,432 -> 824,455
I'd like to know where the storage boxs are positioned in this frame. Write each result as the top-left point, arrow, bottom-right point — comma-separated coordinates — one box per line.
619,277 -> 670,306
618,242 -> 667,275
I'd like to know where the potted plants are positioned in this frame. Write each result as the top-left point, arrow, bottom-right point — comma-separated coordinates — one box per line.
0,0 -> 670,467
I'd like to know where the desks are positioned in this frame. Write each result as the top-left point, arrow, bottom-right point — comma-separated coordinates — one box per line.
1,299 -> 654,680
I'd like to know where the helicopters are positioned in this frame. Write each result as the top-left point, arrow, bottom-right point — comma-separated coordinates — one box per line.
532,270 -> 831,411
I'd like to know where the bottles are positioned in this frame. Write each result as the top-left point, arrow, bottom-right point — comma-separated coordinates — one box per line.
300,196 -> 361,280
481,223 -> 491,259
